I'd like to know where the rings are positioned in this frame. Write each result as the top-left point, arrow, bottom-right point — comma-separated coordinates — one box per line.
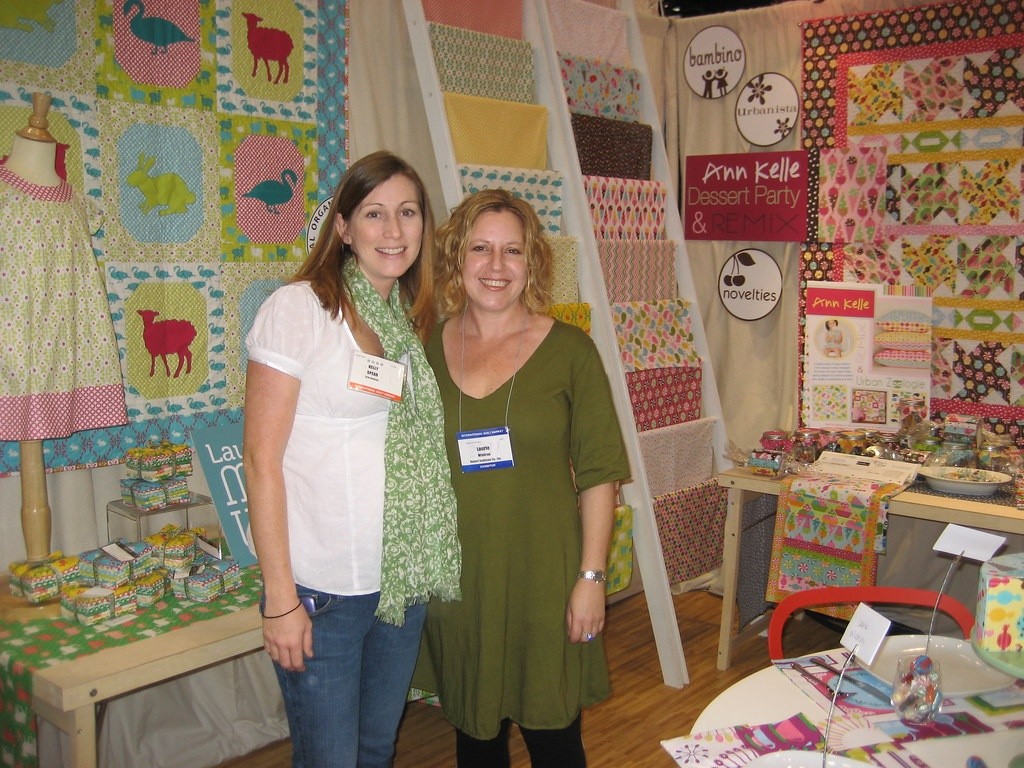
583,632 -> 592,639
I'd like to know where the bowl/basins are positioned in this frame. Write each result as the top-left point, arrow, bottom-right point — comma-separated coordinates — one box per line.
916,466 -> 1013,495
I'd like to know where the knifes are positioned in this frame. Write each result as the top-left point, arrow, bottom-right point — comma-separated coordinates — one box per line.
809,658 -> 892,707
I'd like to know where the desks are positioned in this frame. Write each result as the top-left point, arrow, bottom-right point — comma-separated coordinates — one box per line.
716,465 -> 1024,671
0,524 -> 266,768
688,636 -> 1024,768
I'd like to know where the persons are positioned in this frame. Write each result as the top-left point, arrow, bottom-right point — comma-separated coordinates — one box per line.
824,319 -> 844,358
424,188 -> 630,768
240,148 -> 462,768
1,92 -> 129,561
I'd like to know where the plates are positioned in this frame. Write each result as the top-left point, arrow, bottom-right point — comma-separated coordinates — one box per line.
740,750 -> 879,768
854,635 -> 1016,698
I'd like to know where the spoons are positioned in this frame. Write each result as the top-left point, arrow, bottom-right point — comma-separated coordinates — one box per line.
791,662 -> 856,700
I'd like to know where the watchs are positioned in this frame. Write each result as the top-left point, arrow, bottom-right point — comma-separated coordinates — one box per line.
577,569 -> 607,583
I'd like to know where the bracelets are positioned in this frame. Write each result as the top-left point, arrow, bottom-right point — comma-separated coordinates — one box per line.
262,600 -> 302,618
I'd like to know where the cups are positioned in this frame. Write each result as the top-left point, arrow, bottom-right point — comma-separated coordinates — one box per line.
890,654 -> 944,727
